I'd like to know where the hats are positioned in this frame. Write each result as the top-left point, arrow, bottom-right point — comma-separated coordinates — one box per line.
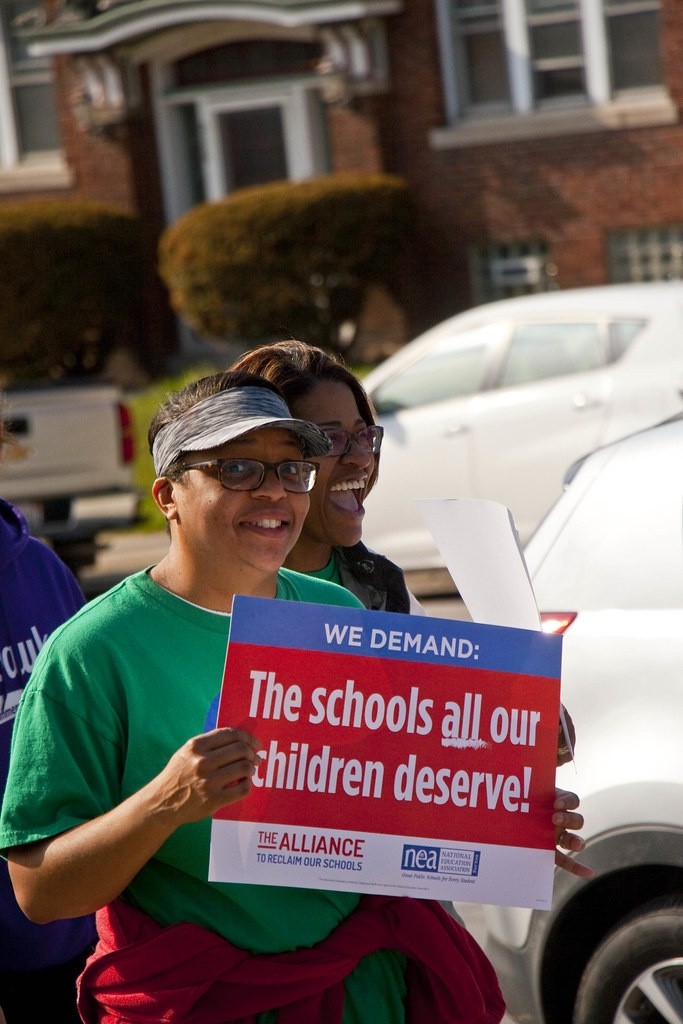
152,385 -> 333,478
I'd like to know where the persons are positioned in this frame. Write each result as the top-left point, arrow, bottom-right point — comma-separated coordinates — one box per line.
0,372 -> 595,1024
230,342 -> 577,768
0,501 -> 98,1024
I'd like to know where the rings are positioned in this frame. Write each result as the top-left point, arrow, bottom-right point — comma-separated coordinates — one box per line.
557,746 -> 570,755
558,829 -> 566,845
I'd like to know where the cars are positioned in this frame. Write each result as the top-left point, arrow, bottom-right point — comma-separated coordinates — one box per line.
474,423 -> 683,1024
364,280 -> 683,580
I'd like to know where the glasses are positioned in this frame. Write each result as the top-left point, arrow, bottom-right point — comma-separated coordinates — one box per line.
322,426 -> 383,457
173,456 -> 321,493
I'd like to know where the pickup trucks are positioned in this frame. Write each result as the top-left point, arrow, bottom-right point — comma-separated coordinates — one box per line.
1,389 -> 148,582
